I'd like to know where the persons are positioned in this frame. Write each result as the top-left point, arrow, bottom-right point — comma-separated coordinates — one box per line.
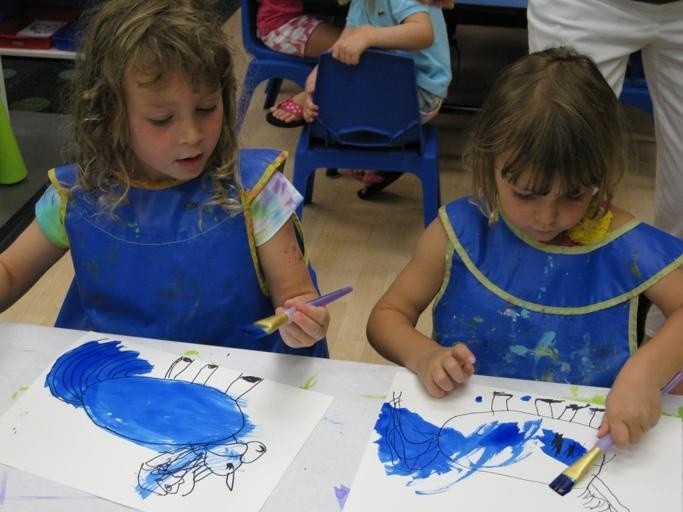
266,0 -> 454,199
256,0 -> 342,55
528,1 -> 683,343
0,0 -> 330,355
367,48 -> 683,448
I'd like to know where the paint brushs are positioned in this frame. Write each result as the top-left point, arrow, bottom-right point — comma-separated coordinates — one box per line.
242,285 -> 353,341
550,372 -> 683,496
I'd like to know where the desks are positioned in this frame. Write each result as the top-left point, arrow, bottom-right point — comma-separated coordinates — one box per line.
0,322 -> 681,512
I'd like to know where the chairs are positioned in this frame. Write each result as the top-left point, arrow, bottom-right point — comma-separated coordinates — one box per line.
291,48 -> 441,233
619,76 -> 653,116
235,0 -> 313,136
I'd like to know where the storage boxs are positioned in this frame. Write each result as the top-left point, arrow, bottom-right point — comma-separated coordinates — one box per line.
0,47 -> 87,117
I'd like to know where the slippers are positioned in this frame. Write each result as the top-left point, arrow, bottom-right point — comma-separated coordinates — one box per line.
356,170 -> 405,198
266,94 -> 306,129
